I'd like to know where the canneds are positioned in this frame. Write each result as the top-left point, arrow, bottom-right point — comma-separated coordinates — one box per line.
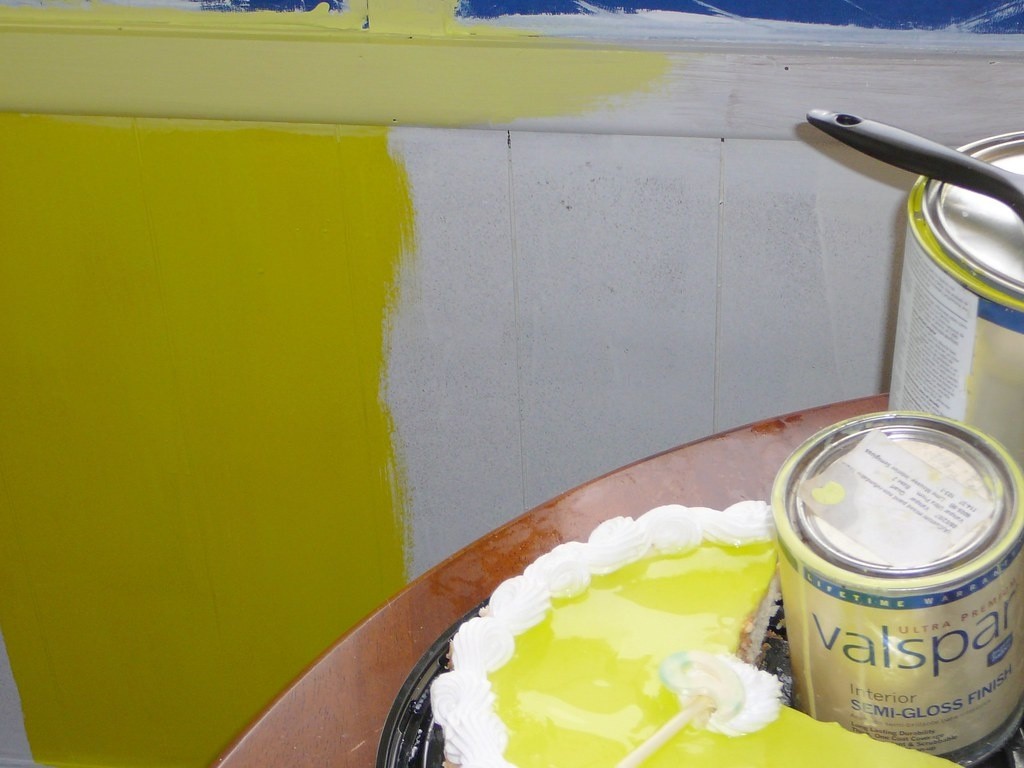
886,128 -> 1024,470
769,410 -> 1024,768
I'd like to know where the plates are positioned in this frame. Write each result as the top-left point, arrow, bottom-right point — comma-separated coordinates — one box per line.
375,596 -> 1023,768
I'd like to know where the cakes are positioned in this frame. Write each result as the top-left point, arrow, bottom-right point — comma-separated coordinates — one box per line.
430,498 -> 972,768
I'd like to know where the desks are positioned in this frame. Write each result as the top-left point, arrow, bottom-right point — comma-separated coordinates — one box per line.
208,393 -> 890,768
